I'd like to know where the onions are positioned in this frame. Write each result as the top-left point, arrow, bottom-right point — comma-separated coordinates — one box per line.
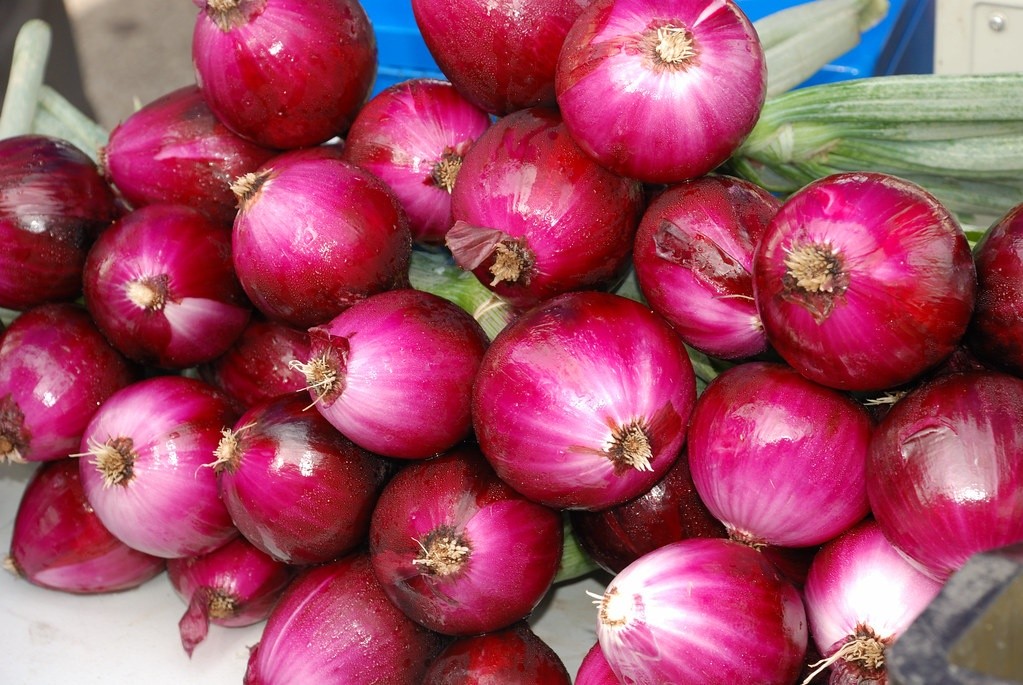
0,0 -> 1023,685
